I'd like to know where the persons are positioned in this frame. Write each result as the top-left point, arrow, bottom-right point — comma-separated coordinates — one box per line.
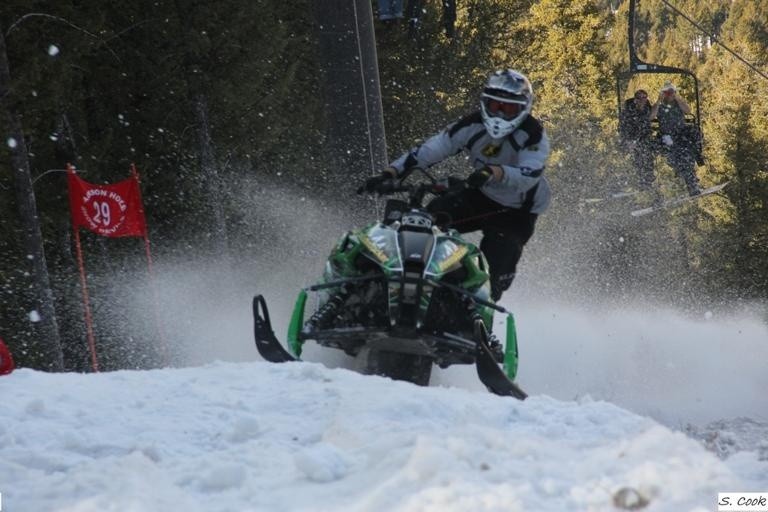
355,65 -> 554,358
645,79 -> 706,196
619,89 -> 659,192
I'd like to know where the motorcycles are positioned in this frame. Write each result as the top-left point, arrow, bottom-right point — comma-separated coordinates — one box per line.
252,178 -> 530,401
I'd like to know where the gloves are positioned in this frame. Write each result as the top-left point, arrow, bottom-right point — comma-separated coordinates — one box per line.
464,164 -> 495,191
356,165 -> 398,197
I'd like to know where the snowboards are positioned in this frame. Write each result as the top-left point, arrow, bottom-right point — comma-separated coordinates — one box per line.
630,181 -> 731,217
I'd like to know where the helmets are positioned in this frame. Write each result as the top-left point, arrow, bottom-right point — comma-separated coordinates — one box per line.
477,66 -> 536,140
